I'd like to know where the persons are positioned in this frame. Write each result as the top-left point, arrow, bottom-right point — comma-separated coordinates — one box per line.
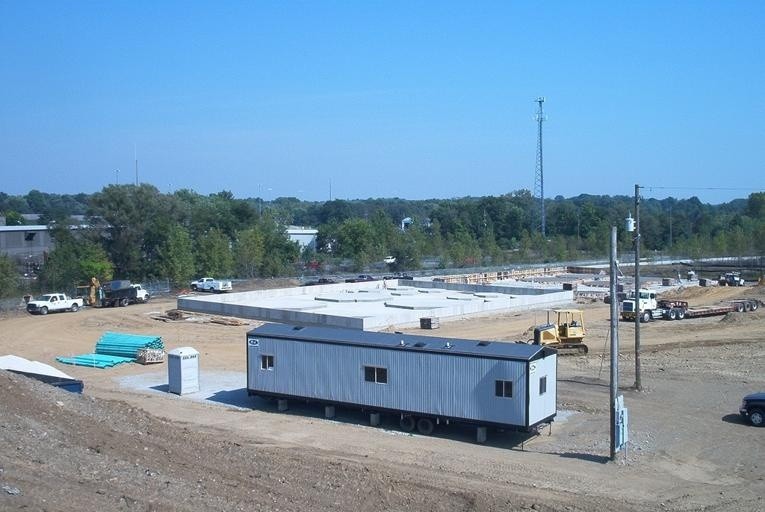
676,270 -> 683,284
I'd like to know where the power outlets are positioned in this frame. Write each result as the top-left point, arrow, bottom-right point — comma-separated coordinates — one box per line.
27,292 -> 84,315
345,273 -> 378,283
306,278 -> 337,286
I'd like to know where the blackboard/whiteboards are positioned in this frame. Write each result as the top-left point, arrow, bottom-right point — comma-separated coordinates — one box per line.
74,277 -> 100,306
516,308 -> 588,359
718,271 -> 745,287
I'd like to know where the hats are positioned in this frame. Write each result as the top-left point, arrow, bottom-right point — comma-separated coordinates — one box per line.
384,255 -> 396,263
384,273 -> 413,281
739,391 -> 765,427
